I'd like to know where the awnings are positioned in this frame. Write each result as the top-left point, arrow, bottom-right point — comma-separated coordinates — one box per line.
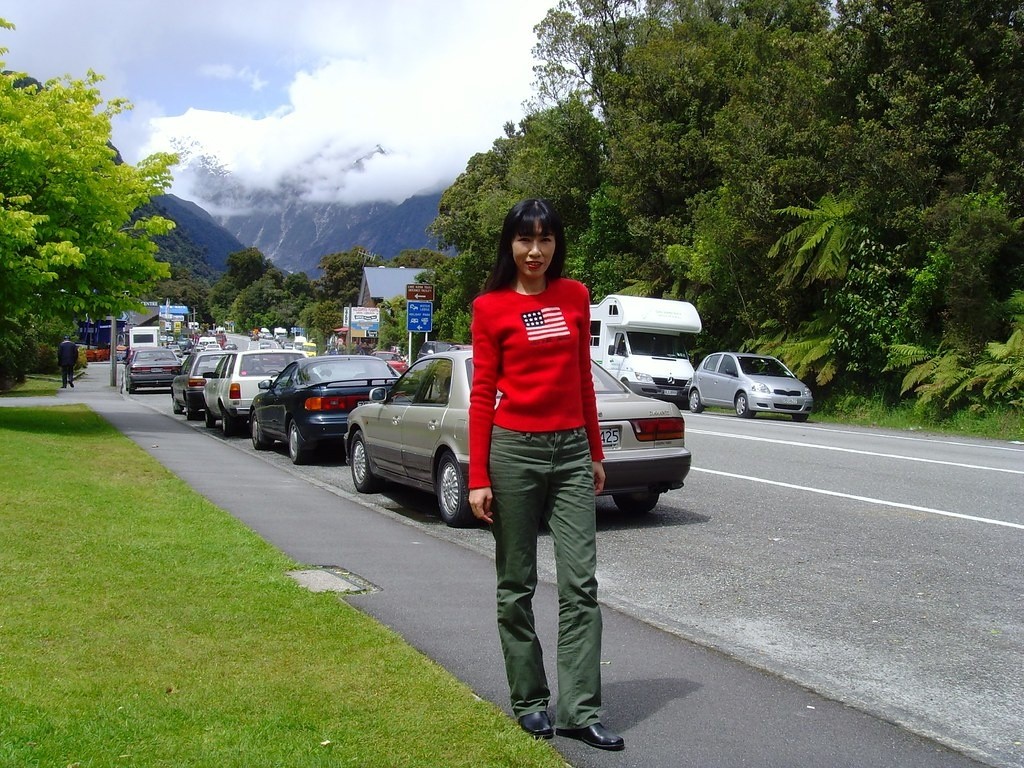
334,328 -> 348,331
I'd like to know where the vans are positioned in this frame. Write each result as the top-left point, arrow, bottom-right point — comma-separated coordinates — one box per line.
127,326 -> 161,350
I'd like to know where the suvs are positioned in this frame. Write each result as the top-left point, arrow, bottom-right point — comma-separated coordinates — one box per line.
416,340 -> 454,360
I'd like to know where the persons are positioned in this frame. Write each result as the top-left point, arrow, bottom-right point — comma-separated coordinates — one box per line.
337,336 -> 344,349
468,199 -> 625,751
58,335 -> 78,388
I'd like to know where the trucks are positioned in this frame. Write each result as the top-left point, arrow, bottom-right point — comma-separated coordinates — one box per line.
590,294 -> 702,410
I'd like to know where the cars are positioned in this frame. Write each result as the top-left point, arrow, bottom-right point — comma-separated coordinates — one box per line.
202,348 -> 322,437
687,351 -> 814,422
170,350 -> 236,421
343,349 -> 691,529
446,345 -> 473,352
249,326 -> 318,358
125,348 -> 185,394
166,327 -> 239,359
248,354 -> 408,466
369,351 -> 409,373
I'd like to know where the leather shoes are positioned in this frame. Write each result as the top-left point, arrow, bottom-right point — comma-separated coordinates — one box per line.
519,712 -> 555,738
557,719 -> 624,749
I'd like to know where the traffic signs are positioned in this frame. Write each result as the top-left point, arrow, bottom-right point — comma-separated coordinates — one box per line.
406,283 -> 435,301
407,301 -> 432,332
